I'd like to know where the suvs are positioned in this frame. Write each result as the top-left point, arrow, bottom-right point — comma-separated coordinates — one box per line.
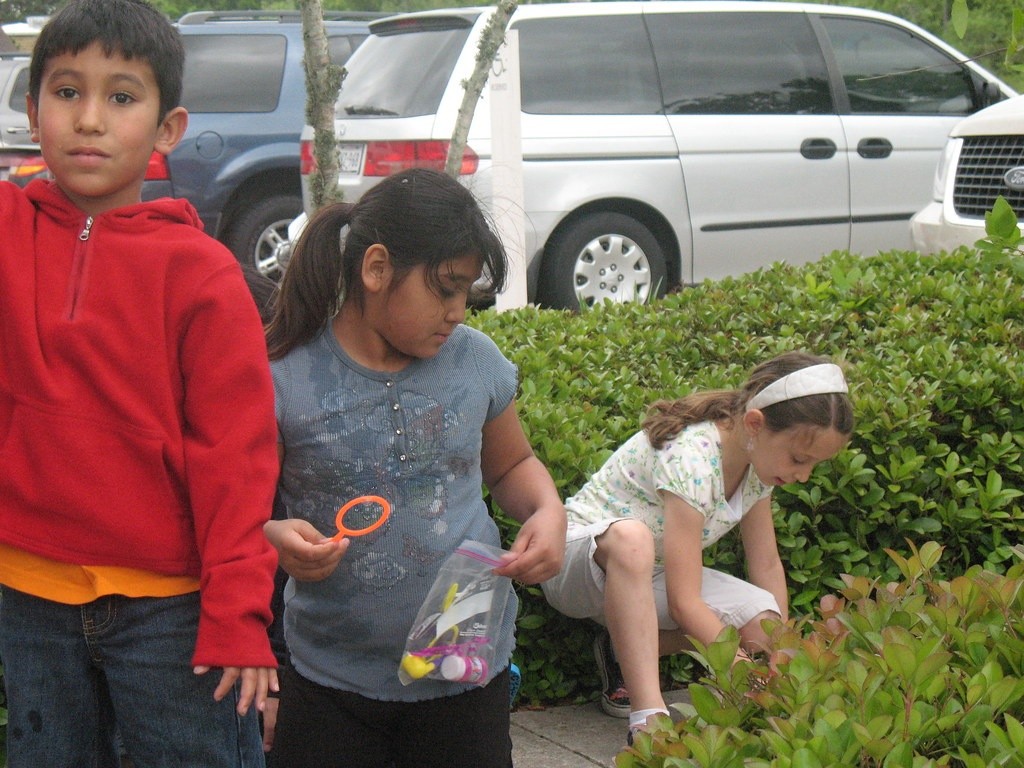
140,9 -> 407,320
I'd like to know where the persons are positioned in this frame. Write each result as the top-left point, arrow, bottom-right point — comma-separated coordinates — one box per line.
0,0 -> 280,768
539,352 -> 852,762
219,259 -> 284,326
265,169 -> 567,767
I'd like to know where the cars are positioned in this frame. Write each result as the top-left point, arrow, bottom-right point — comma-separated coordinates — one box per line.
299,1 -> 1023,314
908,94 -> 1024,256
0,14 -> 53,182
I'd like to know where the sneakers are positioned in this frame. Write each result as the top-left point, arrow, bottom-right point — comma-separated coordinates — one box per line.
627,722 -> 645,748
593,628 -> 632,719
